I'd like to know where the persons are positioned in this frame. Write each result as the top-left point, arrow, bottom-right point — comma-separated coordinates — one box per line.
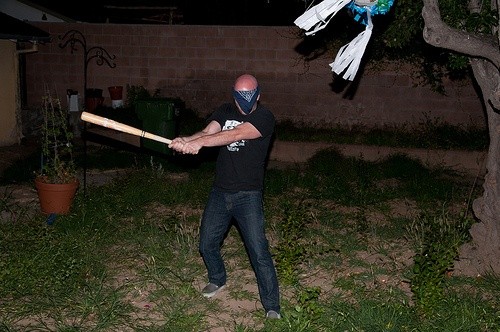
168,75 -> 282,320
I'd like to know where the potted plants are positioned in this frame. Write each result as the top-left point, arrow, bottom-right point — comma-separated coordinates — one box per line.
9,72 -> 123,216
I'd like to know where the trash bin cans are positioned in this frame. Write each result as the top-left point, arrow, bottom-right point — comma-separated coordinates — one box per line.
136,97 -> 178,153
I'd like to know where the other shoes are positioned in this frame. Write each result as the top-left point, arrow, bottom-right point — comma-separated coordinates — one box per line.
201,281 -> 226,298
262,310 -> 281,324
